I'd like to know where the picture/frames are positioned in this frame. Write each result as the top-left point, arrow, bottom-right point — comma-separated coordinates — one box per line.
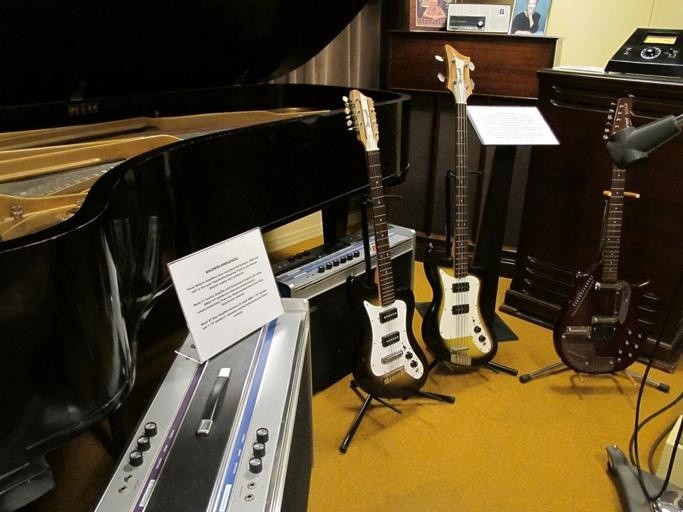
508,0 -> 553,36
410,0 -> 463,32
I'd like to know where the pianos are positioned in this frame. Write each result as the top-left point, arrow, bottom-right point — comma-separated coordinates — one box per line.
0,0 -> 412,511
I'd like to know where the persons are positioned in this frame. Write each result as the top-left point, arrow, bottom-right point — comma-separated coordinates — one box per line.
510,0 -> 542,36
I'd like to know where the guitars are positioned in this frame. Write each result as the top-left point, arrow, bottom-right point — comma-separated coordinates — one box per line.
342,90 -> 429,398
421,44 -> 498,367
553,97 -> 648,373
